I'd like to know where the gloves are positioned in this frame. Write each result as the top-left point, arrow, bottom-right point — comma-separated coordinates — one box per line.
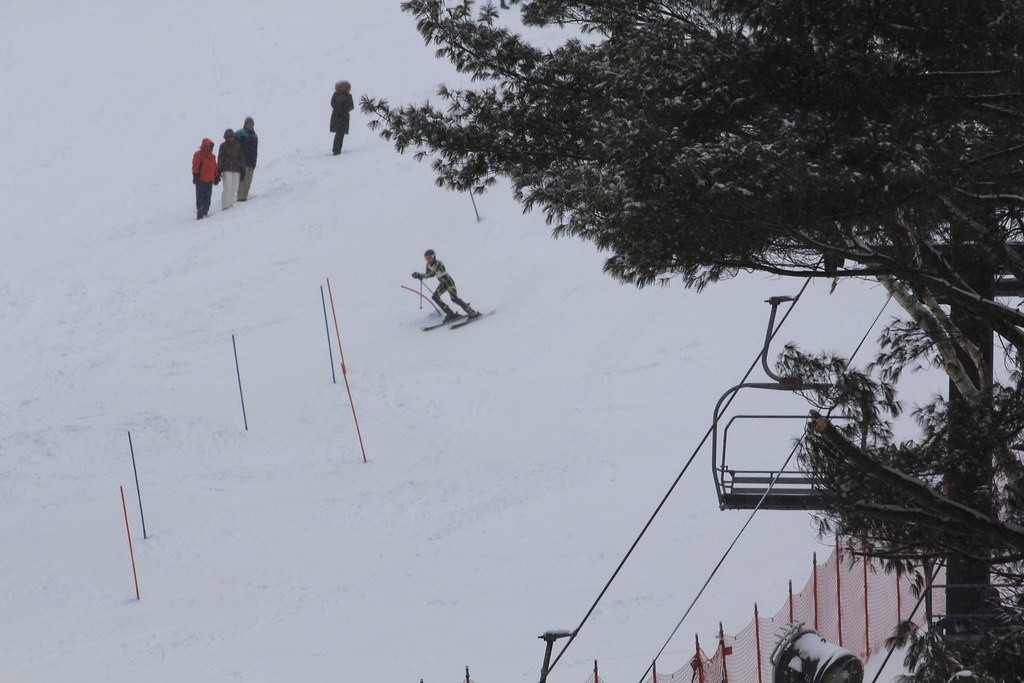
214,176 -> 218,185
192,174 -> 198,184
411,271 -> 423,281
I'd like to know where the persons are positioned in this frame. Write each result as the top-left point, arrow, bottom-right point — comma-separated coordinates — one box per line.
329,81 -> 354,156
192,117 -> 258,221
412,249 -> 478,320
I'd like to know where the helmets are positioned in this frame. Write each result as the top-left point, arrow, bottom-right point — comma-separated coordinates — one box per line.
424,250 -> 436,263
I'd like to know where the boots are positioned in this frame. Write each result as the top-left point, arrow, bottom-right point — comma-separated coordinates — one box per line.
463,303 -> 477,321
443,305 -> 456,323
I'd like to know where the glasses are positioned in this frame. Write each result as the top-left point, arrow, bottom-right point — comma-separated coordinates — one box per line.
424,255 -> 434,260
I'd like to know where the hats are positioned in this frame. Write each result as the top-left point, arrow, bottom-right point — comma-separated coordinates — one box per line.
244,116 -> 254,126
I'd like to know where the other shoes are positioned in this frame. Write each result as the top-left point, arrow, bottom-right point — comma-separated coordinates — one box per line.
222,205 -> 234,211
237,199 -> 247,202
197,215 -> 208,221
333,152 -> 341,155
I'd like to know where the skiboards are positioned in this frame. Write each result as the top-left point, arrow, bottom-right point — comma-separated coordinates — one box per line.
420,310 -> 496,332
